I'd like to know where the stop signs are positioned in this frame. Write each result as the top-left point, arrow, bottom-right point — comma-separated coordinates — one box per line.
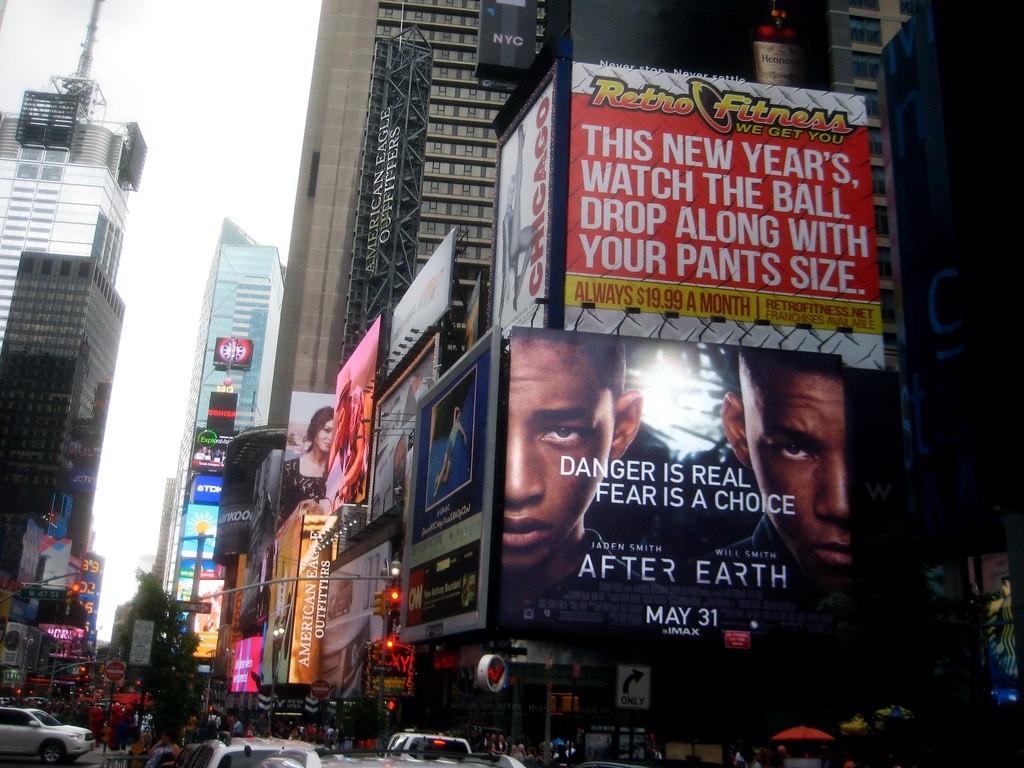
310,679 -> 330,699
104,661 -> 127,683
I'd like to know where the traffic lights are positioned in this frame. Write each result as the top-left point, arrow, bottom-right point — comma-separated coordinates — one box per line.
372,587 -> 403,619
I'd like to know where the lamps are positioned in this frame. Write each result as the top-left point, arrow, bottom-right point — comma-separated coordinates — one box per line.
355,412 -> 416,439
530,297 -> 853,352
316,512 -> 380,550
362,328 -> 425,393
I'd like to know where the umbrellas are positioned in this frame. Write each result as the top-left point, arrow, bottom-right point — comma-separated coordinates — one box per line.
772,725 -> 835,740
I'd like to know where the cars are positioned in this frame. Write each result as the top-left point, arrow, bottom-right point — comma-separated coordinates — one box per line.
178,734 -> 528,767
0,707 -> 97,763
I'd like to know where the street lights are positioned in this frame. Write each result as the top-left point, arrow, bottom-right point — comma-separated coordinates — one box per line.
271,623 -> 287,734
377,551 -> 406,742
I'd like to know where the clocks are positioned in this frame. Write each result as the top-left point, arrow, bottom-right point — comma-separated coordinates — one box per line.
220,340 -> 246,362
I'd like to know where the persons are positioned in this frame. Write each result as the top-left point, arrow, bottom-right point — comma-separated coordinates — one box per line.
433,406 -> 466,497
731,739 -> 829,768
277,406 -> 334,531
0,697 -> 91,727
144,730 -> 182,768
694,346 -> 854,590
633,741 -> 662,759
184,709 -> 292,744
328,367 -> 368,504
469,730 -> 576,768
501,326 -> 688,623
289,722 -> 353,750
194,447 -> 226,462
